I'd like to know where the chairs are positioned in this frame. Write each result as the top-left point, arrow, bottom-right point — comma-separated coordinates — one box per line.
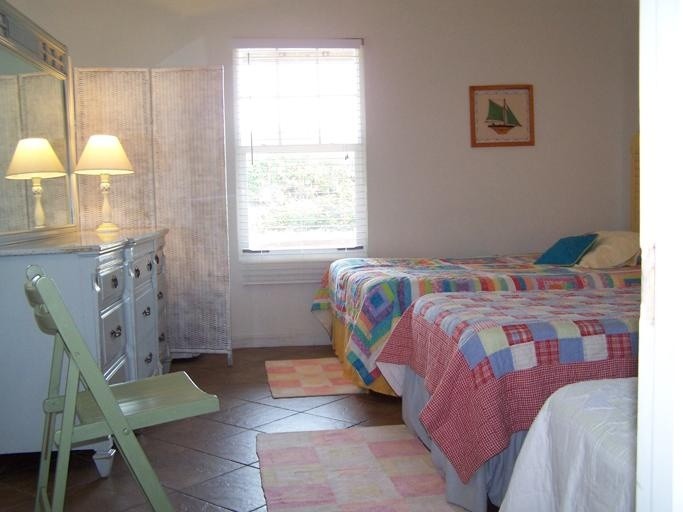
20,262 -> 219,511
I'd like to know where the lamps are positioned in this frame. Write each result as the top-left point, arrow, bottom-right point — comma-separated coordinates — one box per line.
72,133 -> 135,234
5,136 -> 68,228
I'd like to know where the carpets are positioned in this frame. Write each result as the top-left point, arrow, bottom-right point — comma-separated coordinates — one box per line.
254,424 -> 467,512
264,357 -> 371,398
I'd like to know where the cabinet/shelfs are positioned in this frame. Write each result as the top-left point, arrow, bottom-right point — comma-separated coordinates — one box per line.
0,227 -> 172,480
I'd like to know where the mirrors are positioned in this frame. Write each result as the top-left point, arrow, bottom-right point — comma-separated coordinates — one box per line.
0,2 -> 78,246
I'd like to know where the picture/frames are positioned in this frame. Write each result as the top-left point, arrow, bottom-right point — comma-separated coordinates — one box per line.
467,83 -> 535,148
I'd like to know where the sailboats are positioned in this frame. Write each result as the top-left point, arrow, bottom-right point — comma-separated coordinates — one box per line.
483,98 -> 522,135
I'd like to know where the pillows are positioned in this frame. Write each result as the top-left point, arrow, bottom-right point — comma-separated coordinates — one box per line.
530,230 -> 641,270
